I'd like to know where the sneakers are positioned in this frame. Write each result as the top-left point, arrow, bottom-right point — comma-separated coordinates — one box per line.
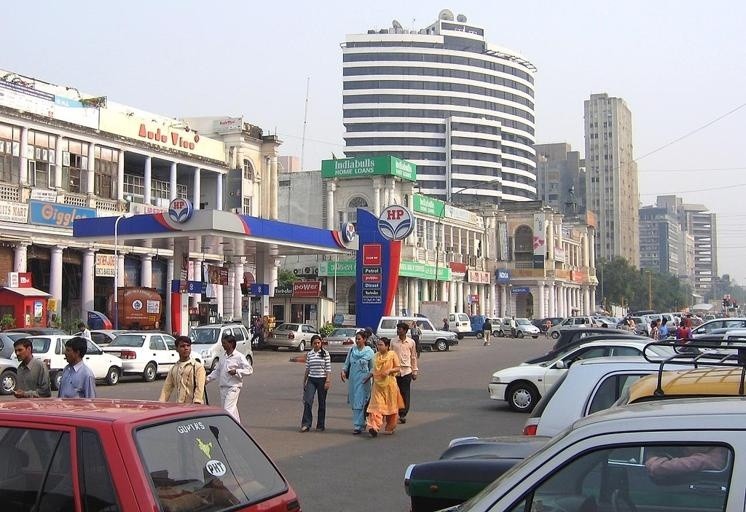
299,418 -> 406,437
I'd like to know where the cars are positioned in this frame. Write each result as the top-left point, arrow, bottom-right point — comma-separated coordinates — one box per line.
546,316 -> 594,339
321,327 -> 365,362
678,337 -> 746,353
268,322 -> 321,351
489,340 -> 693,413
186,323 -> 253,373
405,435 -> 555,511
522,357 -> 745,440
434,397 -> 745,511
711,328 -> 746,335
536,317 -> 564,331
595,310 -> 727,337
546,328 -> 635,354
526,334 -> 679,364
489,318 -> 503,338
0,326 -> 204,394
501,318 -> 541,338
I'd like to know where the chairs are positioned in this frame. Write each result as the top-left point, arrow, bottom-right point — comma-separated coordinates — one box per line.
32,435 -> 108,512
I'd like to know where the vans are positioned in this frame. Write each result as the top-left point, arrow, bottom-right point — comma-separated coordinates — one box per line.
449,312 -> 472,340
691,318 -> 746,338
458,316 -> 488,339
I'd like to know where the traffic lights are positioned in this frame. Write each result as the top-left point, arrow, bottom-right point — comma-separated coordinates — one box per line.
733,300 -> 736,308
722,299 -> 726,306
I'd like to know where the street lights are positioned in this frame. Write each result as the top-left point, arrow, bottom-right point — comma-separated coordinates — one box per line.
434,177 -> 501,302
601,262 -> 608,300
646,270 -> 652,311
113,212 -> 135,329
206,424 -> 249,500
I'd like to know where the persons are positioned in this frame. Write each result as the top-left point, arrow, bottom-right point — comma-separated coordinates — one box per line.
601,313 -> 703,343
509,316 -> 517,339
482,318 -> 492,346
387,323 -> 418,424
204,335 -> 253,424
251,319 -> 260,342
56,336 -> 97,398
440,318 -> 449,351
9,338 -> 51,397
157,336 -> 205,405
340,330 -> 375,434
76,322 -> 92,341
361,337 -> 405,438
410,321 -> 422,359
299,335 -> 331,433
546,318 -> 554,329
645,446 -> 726,479
46,313 -> 59,329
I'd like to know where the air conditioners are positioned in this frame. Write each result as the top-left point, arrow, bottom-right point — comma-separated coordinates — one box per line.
294,266 -> 318,276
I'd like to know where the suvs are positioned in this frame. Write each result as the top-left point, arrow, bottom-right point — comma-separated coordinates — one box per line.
0,398 -> 302,511
611,341 -> 746,406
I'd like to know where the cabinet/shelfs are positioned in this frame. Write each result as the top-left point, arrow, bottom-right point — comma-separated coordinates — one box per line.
197,301 -> 218,326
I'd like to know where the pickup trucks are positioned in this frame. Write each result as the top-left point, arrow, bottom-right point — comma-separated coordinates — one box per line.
377,315 -> 459,352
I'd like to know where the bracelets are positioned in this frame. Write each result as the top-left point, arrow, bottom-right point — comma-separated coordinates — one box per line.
324,380 -> 329,384
